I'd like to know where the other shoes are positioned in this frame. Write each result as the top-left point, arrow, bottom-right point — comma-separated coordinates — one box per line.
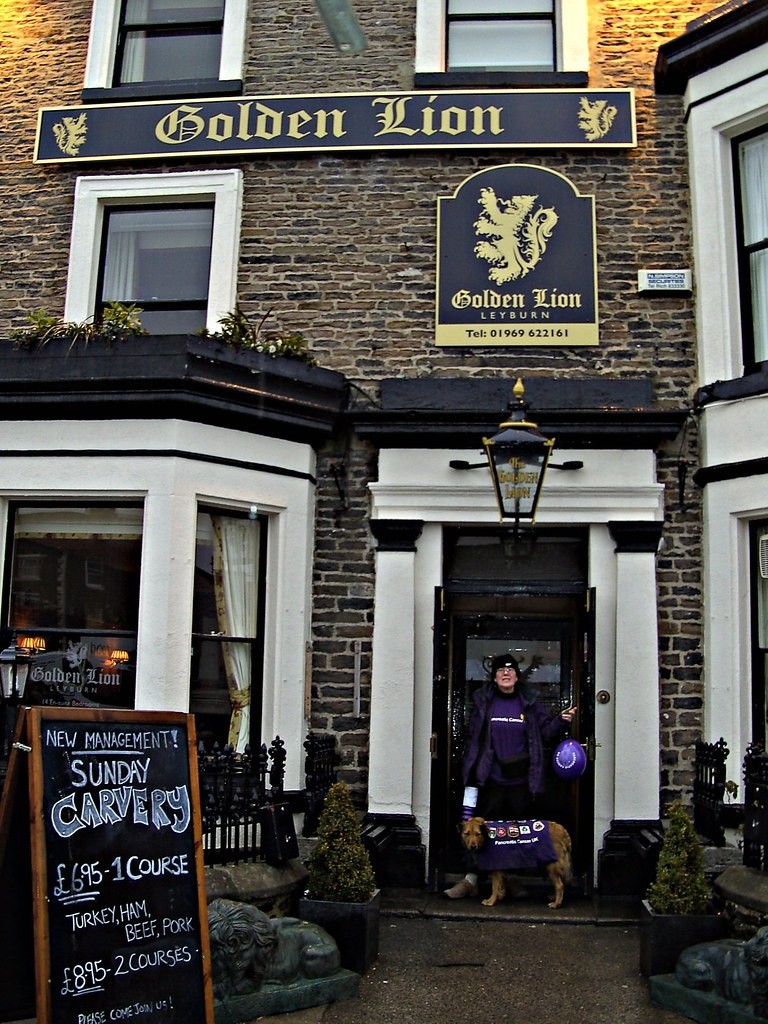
443,879 -> 479,898
508,878 -> 527,897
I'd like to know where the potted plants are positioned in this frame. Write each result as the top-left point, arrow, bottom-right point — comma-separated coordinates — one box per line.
299,780 -> 381,975
639,802 -> 713,988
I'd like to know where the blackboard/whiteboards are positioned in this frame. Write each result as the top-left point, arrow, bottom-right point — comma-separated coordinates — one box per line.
27,704 -> 217,1024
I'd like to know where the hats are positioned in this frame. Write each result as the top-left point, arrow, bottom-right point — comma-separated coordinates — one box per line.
492,654 -> 518,672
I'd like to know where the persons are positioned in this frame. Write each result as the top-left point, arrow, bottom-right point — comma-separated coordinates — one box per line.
444,653 -> 579,899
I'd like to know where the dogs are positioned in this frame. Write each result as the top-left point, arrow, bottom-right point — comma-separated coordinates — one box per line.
456,817 -> 572,908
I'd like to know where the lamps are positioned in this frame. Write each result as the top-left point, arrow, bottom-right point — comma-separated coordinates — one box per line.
21,638 -> 45,654
0,646 -> 36,706
448,379 -> 584,561
107,650 -> 129,672
